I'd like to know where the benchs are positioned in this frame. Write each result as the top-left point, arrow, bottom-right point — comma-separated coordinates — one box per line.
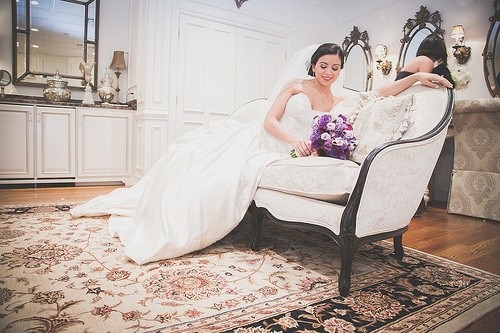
246,83 -> 454,295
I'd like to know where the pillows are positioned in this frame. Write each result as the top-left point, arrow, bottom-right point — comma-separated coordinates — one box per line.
347,92 -> 416,165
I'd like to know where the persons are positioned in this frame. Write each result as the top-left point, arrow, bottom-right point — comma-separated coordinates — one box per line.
392,34 -> 448,217
70,31 -> 453,266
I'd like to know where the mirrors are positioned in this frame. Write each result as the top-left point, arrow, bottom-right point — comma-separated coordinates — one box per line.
397,5 -> 444,74
340,25 -> 373,92
482,0 -> 500,98
11,0 -> 100,90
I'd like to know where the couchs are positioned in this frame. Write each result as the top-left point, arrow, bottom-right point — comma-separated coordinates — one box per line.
447,98 -> 500,221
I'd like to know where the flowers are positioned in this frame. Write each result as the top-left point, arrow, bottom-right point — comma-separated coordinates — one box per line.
290,113 -> 357,159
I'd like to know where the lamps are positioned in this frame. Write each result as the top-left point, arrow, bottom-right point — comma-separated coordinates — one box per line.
375,44 -> 392,75
451,26 -> 471,63
107,51 -> 126,106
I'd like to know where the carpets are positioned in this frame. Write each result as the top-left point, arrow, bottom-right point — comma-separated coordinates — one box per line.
0,200 -> 500,333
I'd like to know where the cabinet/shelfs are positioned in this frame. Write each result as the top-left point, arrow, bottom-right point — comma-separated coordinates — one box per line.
0,103 -> 136,187
